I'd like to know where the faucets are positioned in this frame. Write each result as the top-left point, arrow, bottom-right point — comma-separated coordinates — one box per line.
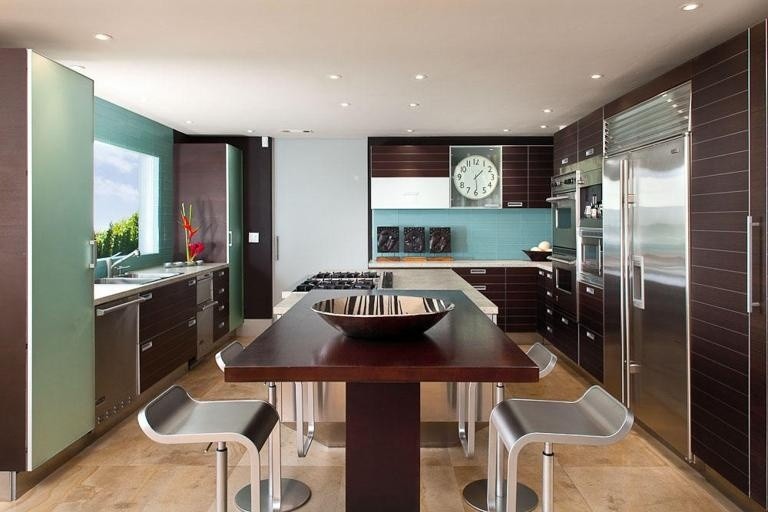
105,248 -> 140,278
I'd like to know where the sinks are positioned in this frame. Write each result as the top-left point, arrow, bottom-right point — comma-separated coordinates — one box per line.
95,277 -> 161,285
114,272 -> 182,279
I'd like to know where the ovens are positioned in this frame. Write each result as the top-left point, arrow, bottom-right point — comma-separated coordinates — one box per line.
546,171 -> 581,256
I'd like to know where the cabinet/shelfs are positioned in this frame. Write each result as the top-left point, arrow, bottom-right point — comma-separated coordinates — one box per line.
371,258 -> 539,333
94,266 -> 230,437
179,143 -> 244,332
539,266 -> 604,384
1,48 -> 95,471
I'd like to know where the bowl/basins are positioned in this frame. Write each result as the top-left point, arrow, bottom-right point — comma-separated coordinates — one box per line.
311,294 -> 456,340
522,249 -> 553,262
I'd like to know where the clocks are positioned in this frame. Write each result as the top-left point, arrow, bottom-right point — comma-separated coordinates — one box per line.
449,146 -> 503,209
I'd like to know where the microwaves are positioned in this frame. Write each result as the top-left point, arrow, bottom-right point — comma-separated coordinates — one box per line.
580,230 -> 603,286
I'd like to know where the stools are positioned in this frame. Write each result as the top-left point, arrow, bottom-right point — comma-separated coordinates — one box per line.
485,384 -> 635,512
215,340 -> 314,460
136,382 -> 282,512
458,340 -> 557,457
